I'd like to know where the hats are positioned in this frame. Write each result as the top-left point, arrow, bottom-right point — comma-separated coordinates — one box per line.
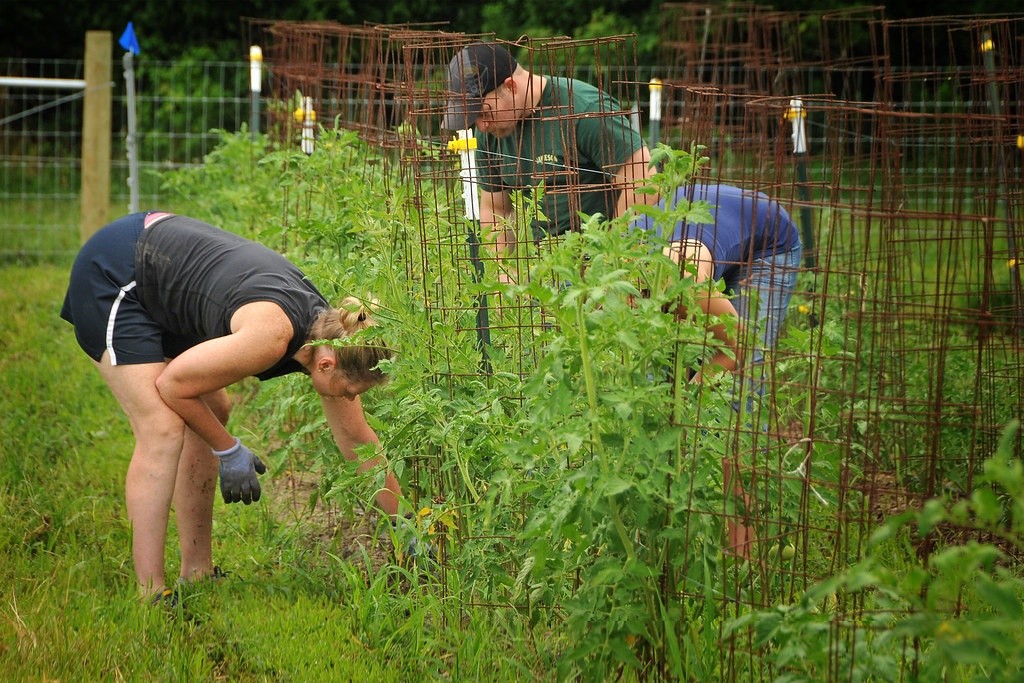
440,43 -> 517,130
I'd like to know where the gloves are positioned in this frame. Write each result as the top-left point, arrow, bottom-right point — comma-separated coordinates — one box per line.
211,436 -> 266,506
390,520 -> 439,558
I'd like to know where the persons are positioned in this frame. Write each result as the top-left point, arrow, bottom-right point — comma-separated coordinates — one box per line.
583,184 -> 801,558
439,43 -> 660,336
61,211 -> 452,601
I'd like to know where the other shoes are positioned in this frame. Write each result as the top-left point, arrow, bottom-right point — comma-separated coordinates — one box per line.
153,588 -> 197,625
